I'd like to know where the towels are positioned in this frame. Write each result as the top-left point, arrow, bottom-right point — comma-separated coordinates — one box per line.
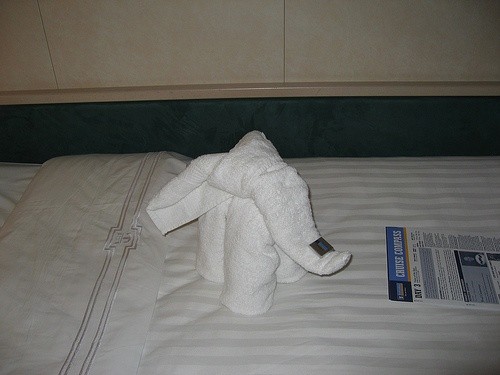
146,131 -> 353,316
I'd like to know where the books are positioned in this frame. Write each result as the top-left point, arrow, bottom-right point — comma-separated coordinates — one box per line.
384,224 -> 500,310
